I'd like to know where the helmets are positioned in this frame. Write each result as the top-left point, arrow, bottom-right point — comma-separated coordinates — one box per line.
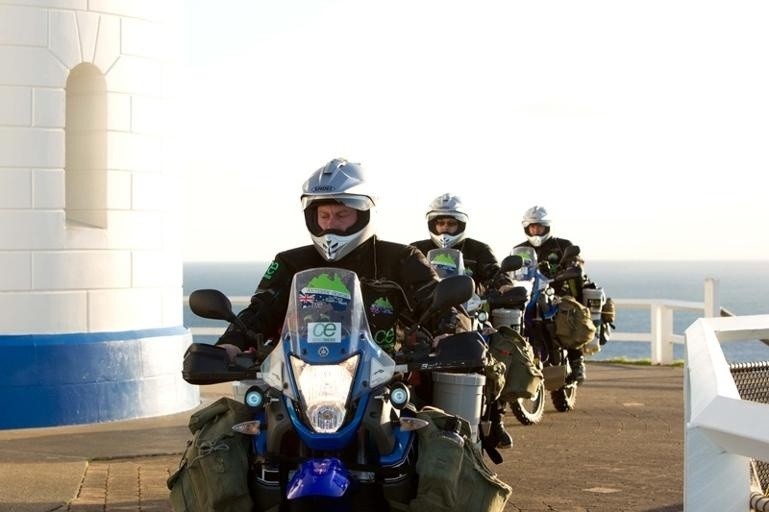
423,192 -> 470,252
521,204 -> 556,246
299,156 -> 379,265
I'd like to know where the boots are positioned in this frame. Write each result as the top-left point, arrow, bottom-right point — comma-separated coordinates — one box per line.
485,404 -> 518,450
565,356 -> 585,383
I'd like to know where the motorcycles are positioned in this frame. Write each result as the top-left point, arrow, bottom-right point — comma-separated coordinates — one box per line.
184,276 -> 492,512
424,250 -> 527,462
510,246 -> 606,426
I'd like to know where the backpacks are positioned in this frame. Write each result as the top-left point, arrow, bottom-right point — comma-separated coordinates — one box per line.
551,296 -> 597,349
409,400 -> 514,512
159,395 -> 256,510
488,325 -> 546,405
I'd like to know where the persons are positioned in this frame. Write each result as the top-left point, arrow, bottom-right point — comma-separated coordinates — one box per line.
216,158 -> 470,410
406,191 -> 516,450
509,205 -> 586,385
